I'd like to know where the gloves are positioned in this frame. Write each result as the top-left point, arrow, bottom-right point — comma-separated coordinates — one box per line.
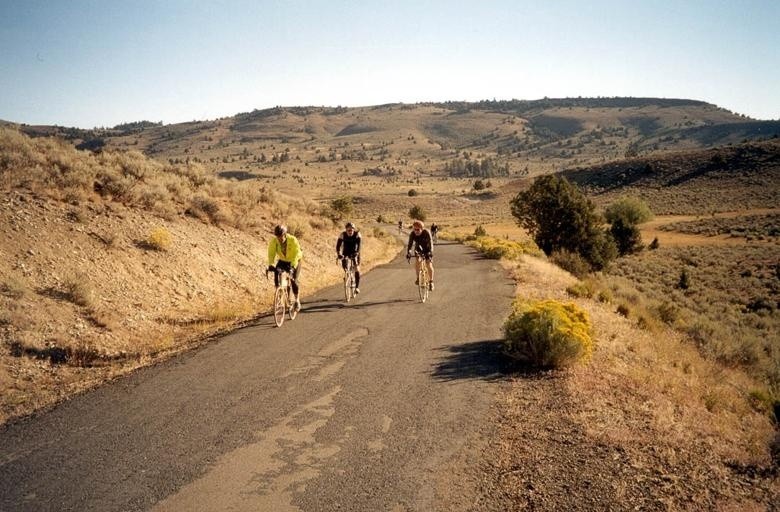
407,254 -> 410,258
338,255 -> 343,259
289,267 -> 295,276
269,266 -> 274,271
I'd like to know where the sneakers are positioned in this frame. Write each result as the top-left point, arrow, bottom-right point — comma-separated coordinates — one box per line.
430,283 -> 434,291
415,277 -> 419,285
294,301 -> 299,312
355,288 -> 360,293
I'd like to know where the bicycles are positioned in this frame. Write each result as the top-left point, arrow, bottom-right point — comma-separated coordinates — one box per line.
432,232 -> 439,245
263,265 -> 300,328
406,251 -> 433,304
334,256 -> 358,303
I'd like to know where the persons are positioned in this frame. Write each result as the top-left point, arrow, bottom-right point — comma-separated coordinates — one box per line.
398,219 -> 403,229
267,225 -> 302,312
431,223 -> 438,239
407,221 -> 435,292
336,222 -> 361,295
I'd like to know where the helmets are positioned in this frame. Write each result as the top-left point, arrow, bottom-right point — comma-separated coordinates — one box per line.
346,222 -> 355,228
275,224 -> 287,235
413,221 -> 424,228
